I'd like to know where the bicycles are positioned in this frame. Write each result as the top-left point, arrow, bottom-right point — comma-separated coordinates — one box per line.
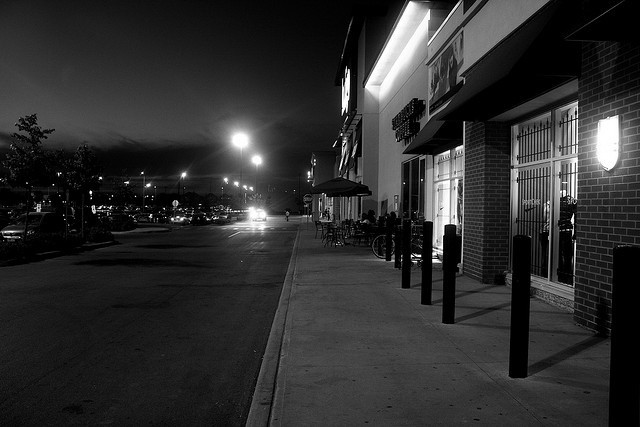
371,221 -> 423,258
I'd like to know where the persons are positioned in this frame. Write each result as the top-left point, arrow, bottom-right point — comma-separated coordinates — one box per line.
285,210 -> 290,221
360,212 -> 373,243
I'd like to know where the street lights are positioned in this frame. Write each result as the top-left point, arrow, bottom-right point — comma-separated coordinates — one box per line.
251,154 -> 263,218
232,132 -> 248,221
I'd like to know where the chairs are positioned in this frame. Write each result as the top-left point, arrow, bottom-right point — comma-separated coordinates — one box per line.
315,220 -> 326,239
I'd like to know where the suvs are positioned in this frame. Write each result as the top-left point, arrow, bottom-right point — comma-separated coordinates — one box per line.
0,212 -> 64,248
